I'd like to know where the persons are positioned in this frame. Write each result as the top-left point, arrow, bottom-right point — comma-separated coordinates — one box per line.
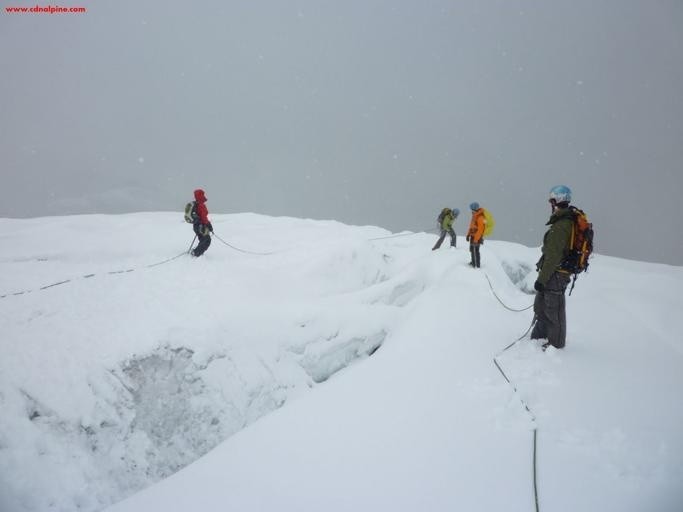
430,207 -> 459,251
529,183 -> 577,353
189,189 -> 213,258
465,200 -> 486,270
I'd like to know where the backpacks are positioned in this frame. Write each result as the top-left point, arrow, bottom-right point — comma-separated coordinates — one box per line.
183,201 -> 200,225
550,209 -> 594,278
481,208 -> 495,237
436,208 -> 451,223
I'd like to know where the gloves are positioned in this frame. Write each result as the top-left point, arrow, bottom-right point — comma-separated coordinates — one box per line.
465,235 -> 469,242
533,280 -> 544,292
207,221 -> 214,232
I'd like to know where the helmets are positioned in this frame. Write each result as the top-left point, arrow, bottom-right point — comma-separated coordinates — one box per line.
469,202 -> 479,210
547,185 -> 572,204
452,208 -> 459,216
